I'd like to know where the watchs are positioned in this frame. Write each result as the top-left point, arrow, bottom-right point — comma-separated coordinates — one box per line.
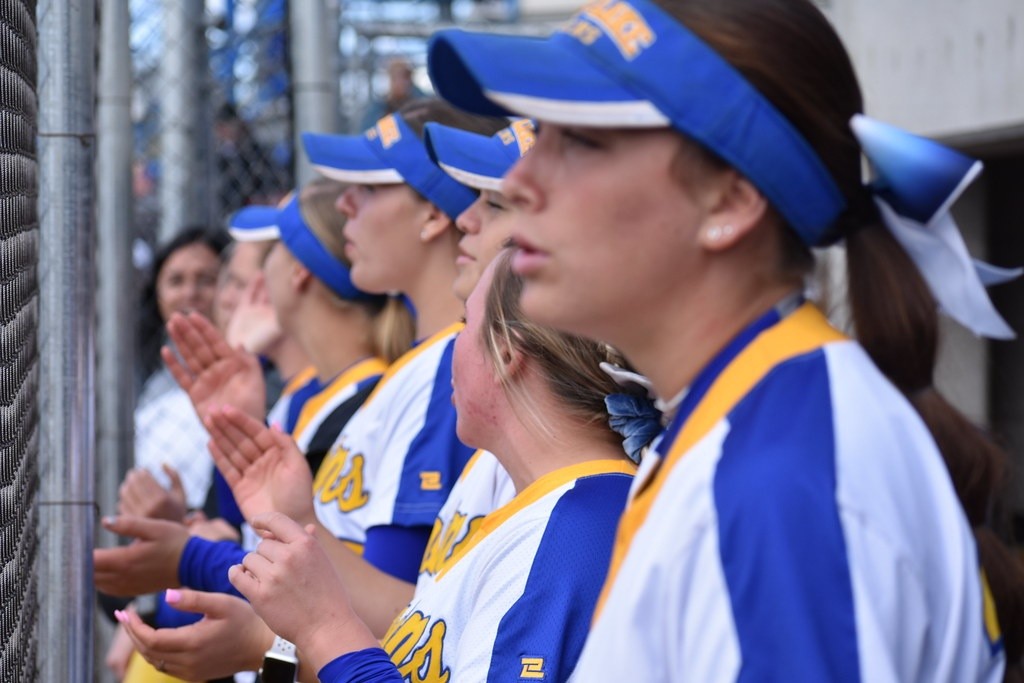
255,634 -> 297,683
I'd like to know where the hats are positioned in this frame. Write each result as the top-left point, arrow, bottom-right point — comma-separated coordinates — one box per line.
425,0 -> 846,245
227,189 -> 355,304
427,116 -> 543,188
303,114 -> 479,220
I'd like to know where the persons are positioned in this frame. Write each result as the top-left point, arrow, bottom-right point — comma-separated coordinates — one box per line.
425,0 -> 1023,683
92,59 -> 664,683
